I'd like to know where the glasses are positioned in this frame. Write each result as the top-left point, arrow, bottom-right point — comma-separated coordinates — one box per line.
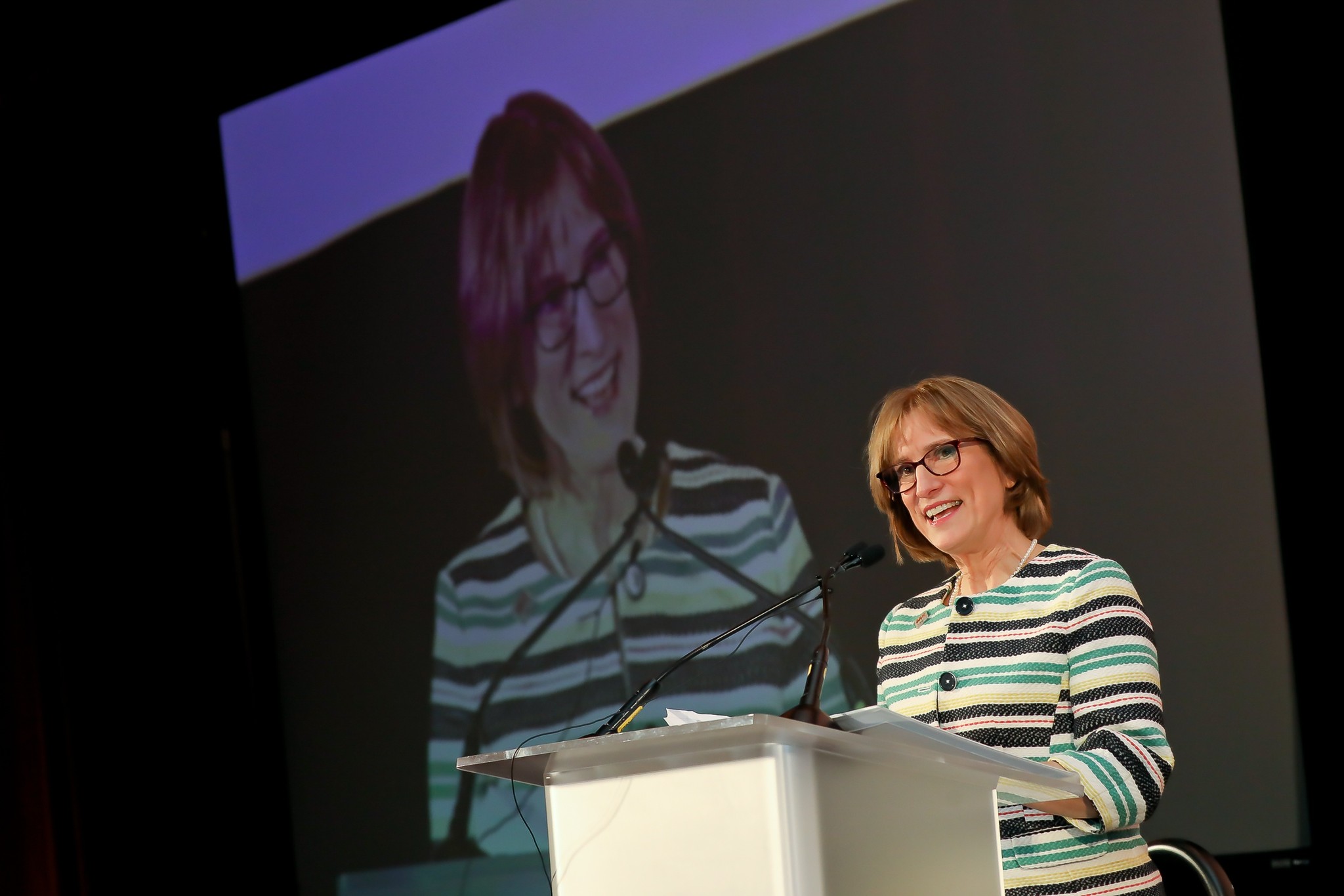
526,223 -> 637,352
875,437 -> 990,494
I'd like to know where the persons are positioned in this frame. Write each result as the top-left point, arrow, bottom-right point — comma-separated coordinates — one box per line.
426,93 -> 851,856
867,377 -> 1175,895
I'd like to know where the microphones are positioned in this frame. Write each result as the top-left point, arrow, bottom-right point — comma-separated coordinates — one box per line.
416,435 -> 875,862
584,541 -> 888,739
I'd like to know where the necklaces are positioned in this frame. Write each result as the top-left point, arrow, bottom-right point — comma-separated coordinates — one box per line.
534,459 -> 667,581
949,538 -> 1038,607
